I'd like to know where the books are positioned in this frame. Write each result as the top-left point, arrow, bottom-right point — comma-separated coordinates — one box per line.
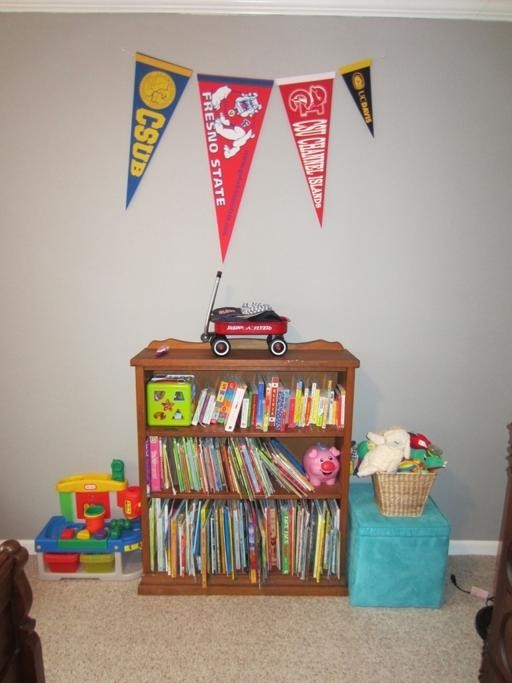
146,497 -> 340,588
191,376 -> 345,432
145,435 -> 315,501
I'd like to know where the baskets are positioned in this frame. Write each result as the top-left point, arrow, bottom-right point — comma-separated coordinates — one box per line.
370,470 -> 437,520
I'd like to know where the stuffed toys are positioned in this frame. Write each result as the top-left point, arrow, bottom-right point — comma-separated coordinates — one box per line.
349,420 -> 449,479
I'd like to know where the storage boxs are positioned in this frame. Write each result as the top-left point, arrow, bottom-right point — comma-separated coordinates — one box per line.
345,482 -> 451,609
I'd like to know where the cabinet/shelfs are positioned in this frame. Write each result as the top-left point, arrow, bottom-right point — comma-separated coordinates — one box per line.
129,338 -> 362,597
